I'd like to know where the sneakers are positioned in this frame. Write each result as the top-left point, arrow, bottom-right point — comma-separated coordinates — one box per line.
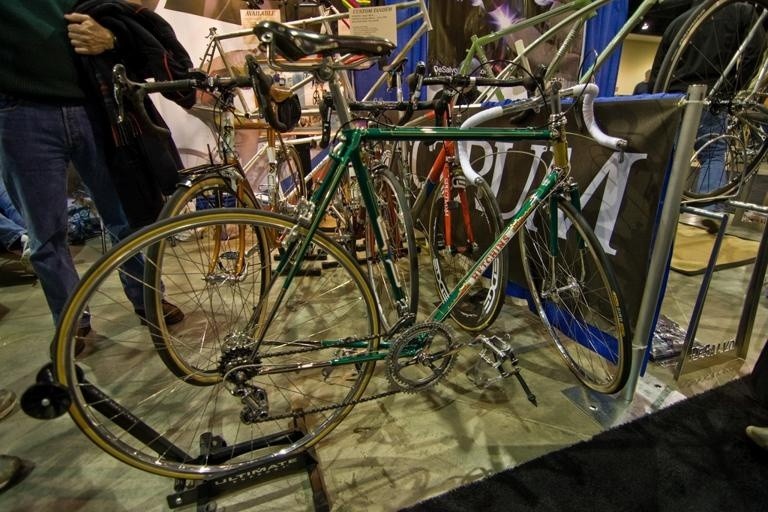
48,319 -> 93,363
0,388 -> 17,420
0,453 -> 23,489
135,299 -> 183,327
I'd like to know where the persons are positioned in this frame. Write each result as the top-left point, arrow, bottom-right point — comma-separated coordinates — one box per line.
0,0 -> 185,361
648,0 -> 766,234
633,70 -> 652,95
0,177 -> 29,257
